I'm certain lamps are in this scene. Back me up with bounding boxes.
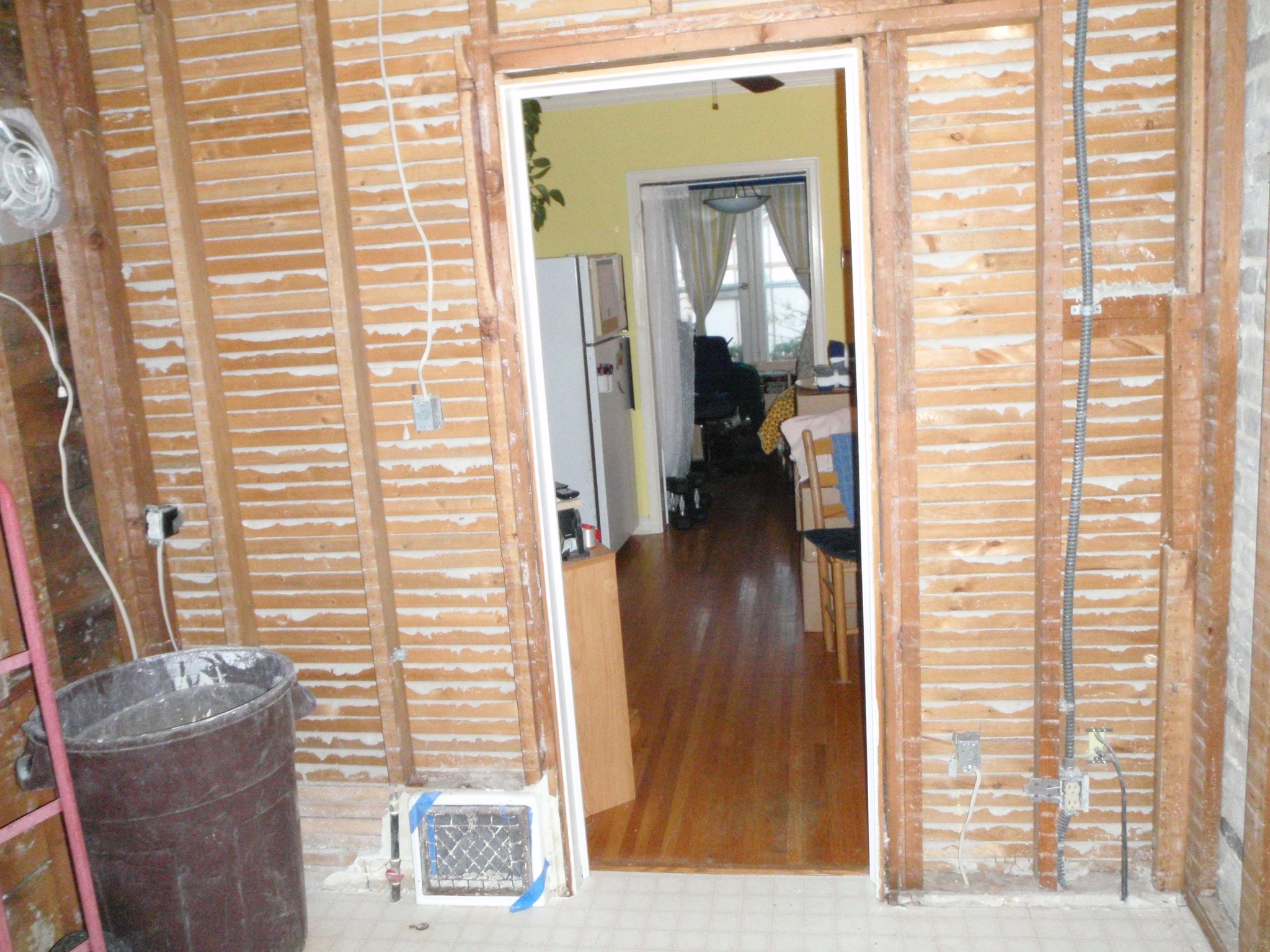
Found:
[703,182,772,214]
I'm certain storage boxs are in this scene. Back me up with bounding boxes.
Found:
[799,483,862,633]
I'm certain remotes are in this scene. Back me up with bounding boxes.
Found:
[556,488,580,501]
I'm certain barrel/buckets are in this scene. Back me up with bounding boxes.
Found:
[16,643,317,950]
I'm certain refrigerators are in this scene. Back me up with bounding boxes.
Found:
[535,251,640,551]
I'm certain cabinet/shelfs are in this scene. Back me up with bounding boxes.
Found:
[562,541,636,819]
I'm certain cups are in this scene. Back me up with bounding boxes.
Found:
[581,524,596,550]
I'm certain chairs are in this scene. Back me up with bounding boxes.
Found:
[802,429,862,680]
[693,335,739,486]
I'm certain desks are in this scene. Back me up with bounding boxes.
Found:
[796,386,857,416]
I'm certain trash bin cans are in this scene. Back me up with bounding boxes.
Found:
[15,645,317,952]
